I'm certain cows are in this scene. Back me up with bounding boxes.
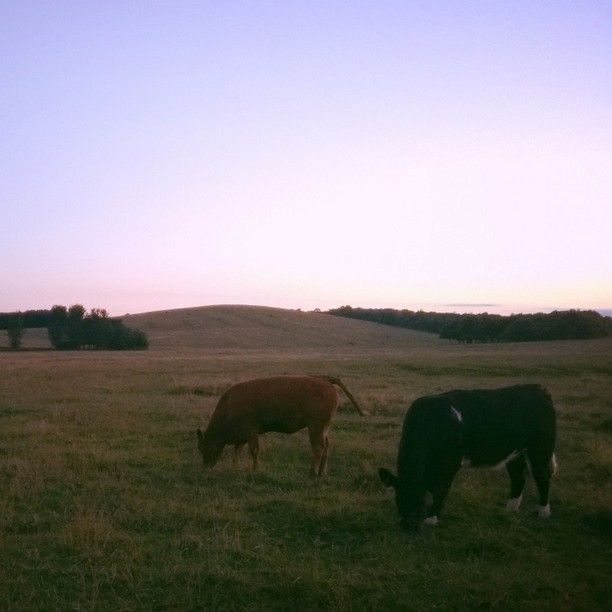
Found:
[381,381,559,527]
[193,371,366,487]
